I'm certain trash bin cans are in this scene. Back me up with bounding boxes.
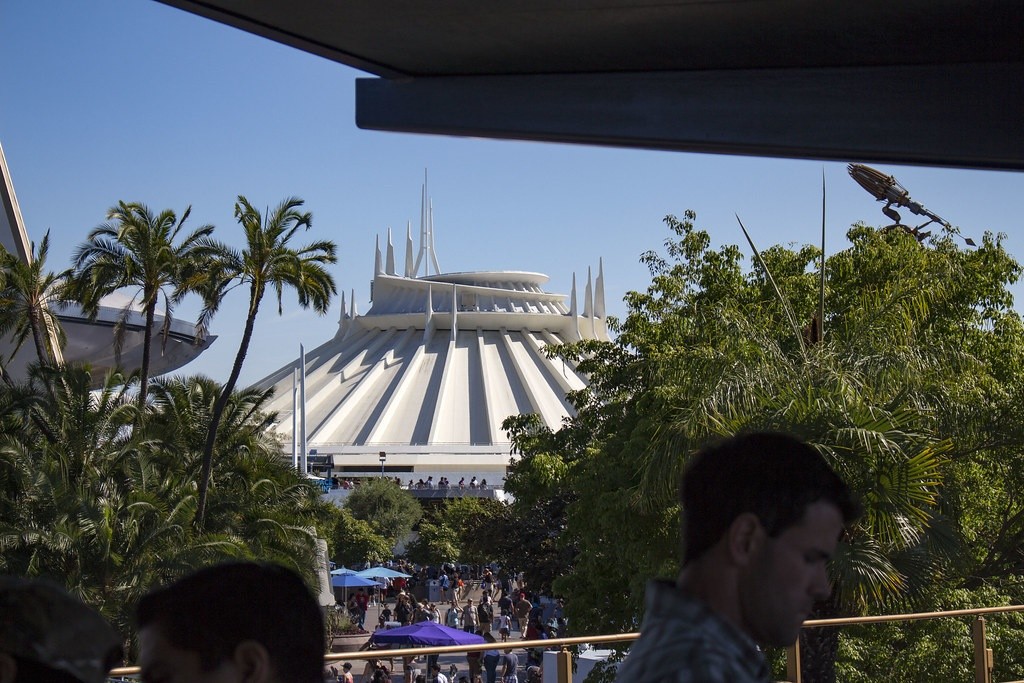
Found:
[428,579,441,602]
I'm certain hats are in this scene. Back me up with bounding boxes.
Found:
[520,593,525,598]
[341,662,352,669]
[0,577,118,683]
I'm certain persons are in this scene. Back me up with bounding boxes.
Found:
[445,599,480,633]
[332,475,488,489]
[375,604,391,631]
[439,571,464,607]
[348,588,368,629]
[133,562,324,682]
[331,663,354,683]
[480,566,510,603]
[616,432,850,683]
[478,589,533,642]
[404,656,417,683]
[393,592,441,627]
[368,577,407,605]
[526,619,550,665]
[432,632,540,683]
[361,660,392,683]
[0,582,120,683]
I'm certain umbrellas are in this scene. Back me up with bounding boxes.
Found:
[332,571,382,601]
[330,565,357,574]
[371,622,482,683]
[359,566,413,577]
